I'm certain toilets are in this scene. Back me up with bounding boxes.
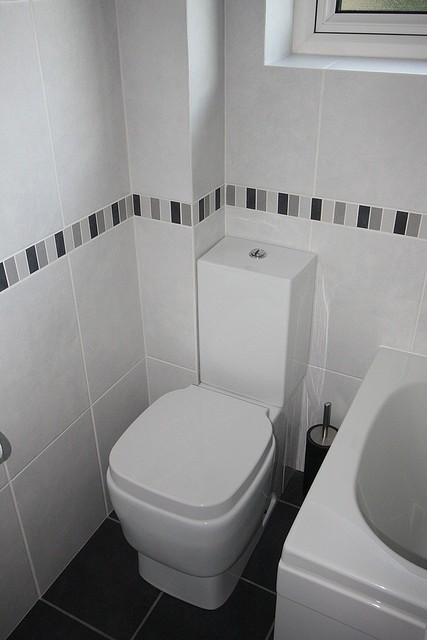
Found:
[106,237,318,610]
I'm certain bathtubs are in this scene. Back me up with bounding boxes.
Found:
[275,348,426,640]
